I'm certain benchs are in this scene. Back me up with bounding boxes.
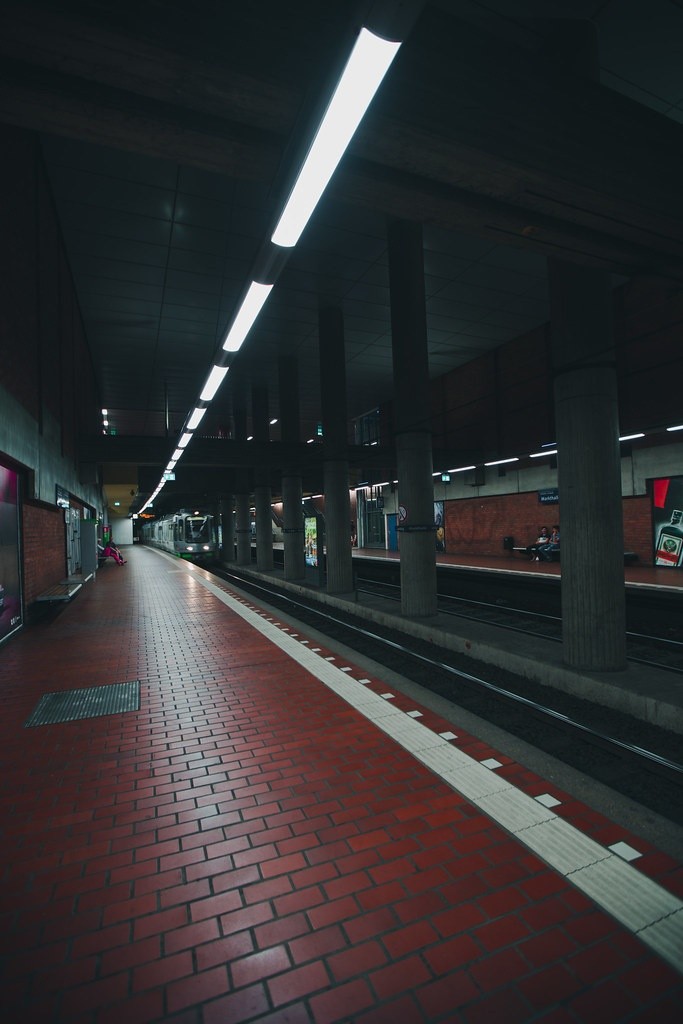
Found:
[59,573,94,584]
[513,547,559,556]
[35,583,83,602]
[623,552,637,562]
[98,555,111,565]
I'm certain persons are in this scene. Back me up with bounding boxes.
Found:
[526,527,550,560]
[98,537,127,565]
[539,525,560,562]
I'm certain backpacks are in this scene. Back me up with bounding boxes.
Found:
[101,546,110,557]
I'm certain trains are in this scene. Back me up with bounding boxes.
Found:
[140,508,212,560]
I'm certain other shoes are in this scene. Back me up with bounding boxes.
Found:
[117,561,124,566]
[353,544,355,547]
[124,560,127,563]
